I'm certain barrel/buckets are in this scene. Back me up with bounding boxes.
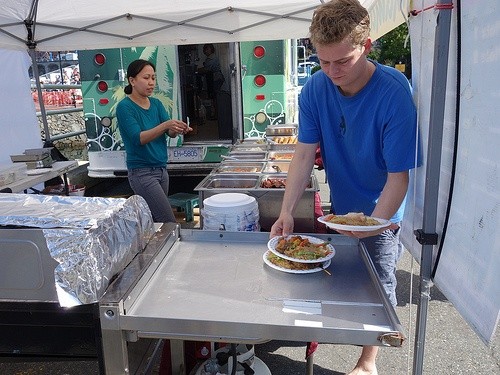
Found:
[201,193,261,232]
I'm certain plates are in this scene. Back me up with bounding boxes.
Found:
[267,234,335,263]
[317,215,391,231]
[264,250,331,273]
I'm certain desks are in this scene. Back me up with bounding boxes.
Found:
[0,161,84,196]
[99,223,407,375]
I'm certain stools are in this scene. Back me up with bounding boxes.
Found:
[167,193,199,222]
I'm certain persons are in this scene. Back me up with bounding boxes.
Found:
[268,0,423,375]
[198,44,226,120]
[115,59,193,222]
